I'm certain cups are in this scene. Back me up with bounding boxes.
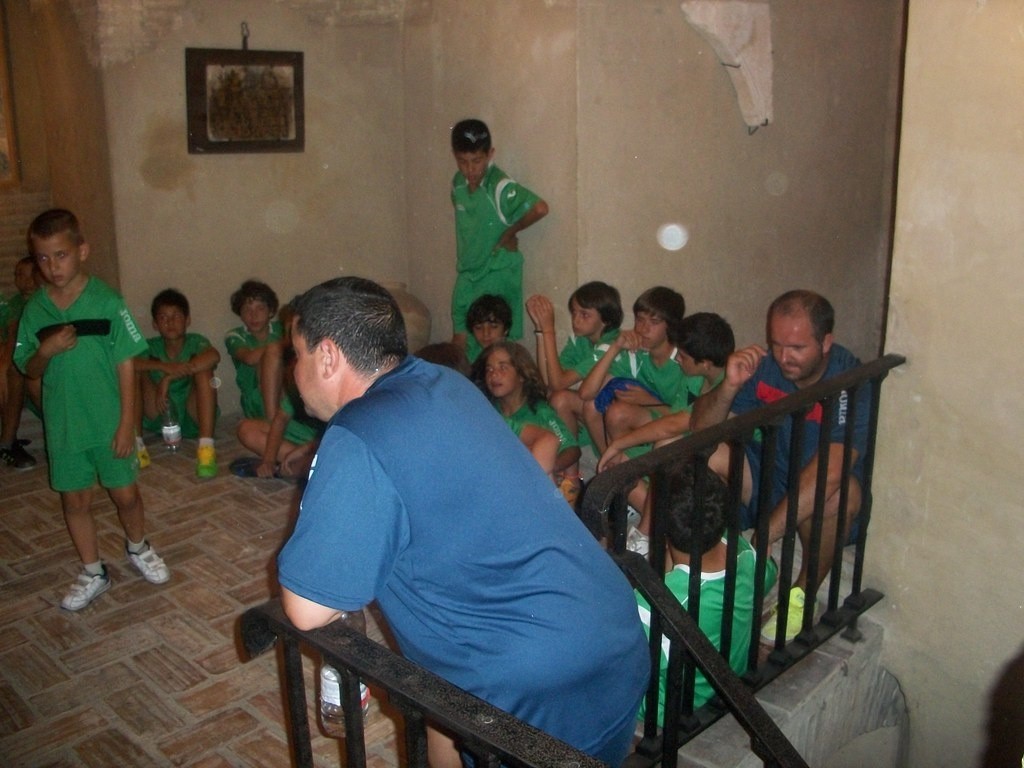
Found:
[196,437,217,479]
[131,430,149,469]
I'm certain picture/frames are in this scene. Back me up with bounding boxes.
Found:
[185,47,306,153]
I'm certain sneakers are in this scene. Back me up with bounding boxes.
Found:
[0,443,38,471]
[556,477,586,504]
[60,565,111,611]
[229,457,286,478]
[132,437,151,467]
[123,539,170,584]
[196,446,219,478]
[625,527,651,558]
[627,504,640,521]
[759,587,819,648]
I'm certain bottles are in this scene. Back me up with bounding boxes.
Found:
[161,402,183,453]
[317,607,369,739]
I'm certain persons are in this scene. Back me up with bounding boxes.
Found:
[625,459,785,730]
[1,256,46,468]
[691,291,873,647]
[468,279,764,564]
[450,118,551,369]
[291,275,654,765]
[128,287,224,480]
[14,207,168,611]
[221,276,328,476]
